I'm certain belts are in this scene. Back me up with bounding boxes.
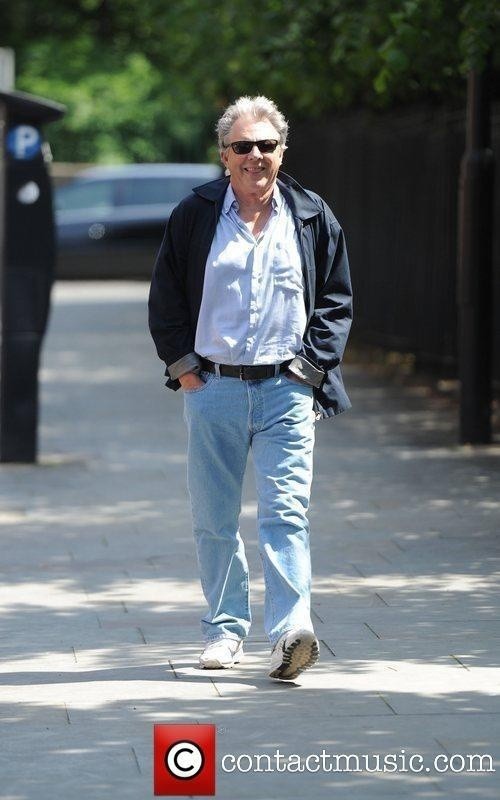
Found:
[198,357,295,381]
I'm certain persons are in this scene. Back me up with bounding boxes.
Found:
[148,96,353,681]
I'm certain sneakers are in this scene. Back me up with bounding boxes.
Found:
[267,628,321,680]
[198,638,245,670]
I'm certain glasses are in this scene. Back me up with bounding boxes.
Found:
[222,138,279,155]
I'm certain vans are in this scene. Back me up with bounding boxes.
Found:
[51,161,224,279]
